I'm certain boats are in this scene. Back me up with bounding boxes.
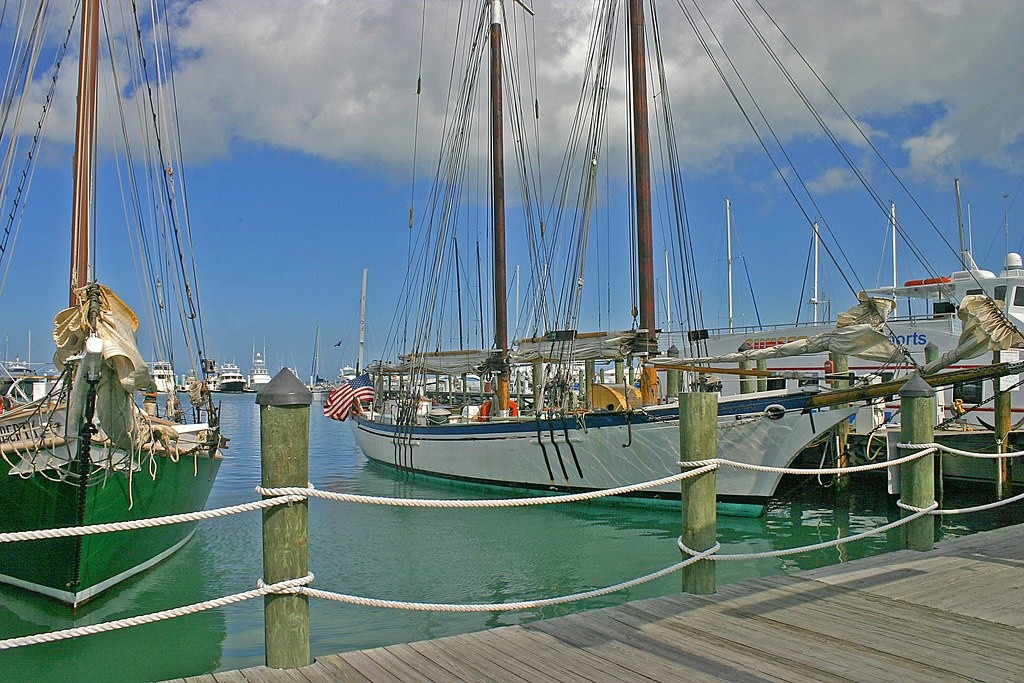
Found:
[242,336,272,393]
[218,355,247,393]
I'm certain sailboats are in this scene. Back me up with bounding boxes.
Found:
[1,1,224,611]
[306,1,1024,519]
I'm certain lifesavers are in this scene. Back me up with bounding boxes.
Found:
[764,403,787,422]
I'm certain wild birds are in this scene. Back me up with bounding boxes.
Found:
[1000,193,1011,199]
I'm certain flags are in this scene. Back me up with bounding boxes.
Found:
[323,373,382,422]
[334,340,342,347]
